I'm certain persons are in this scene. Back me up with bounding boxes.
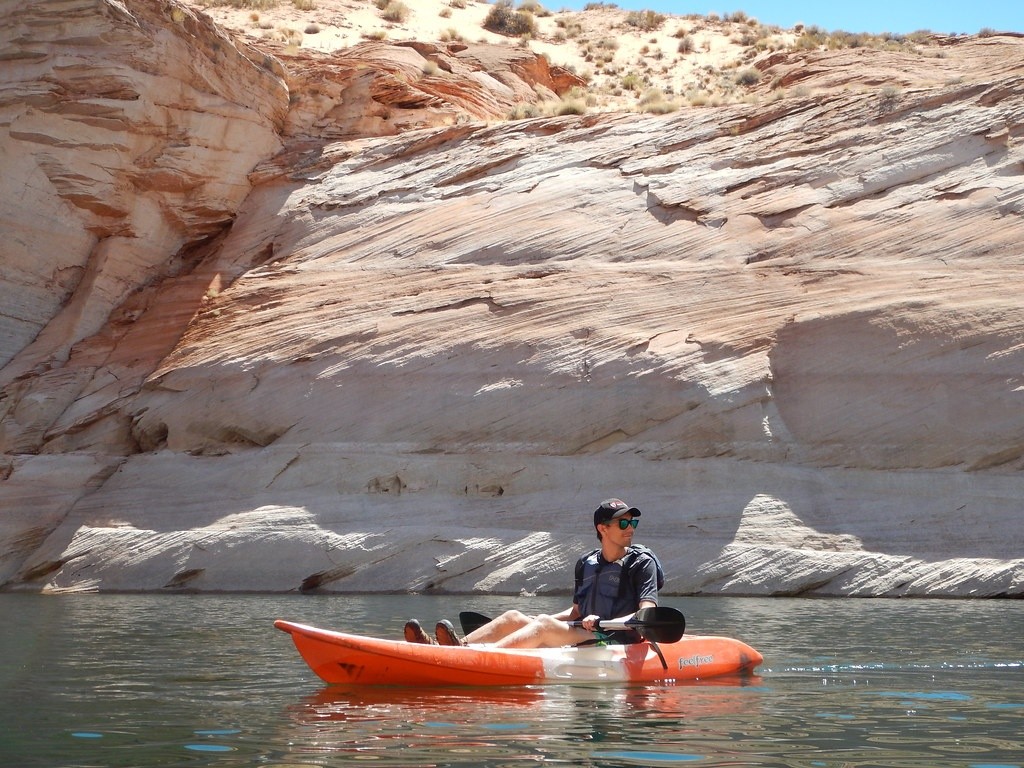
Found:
[403,497,664,648]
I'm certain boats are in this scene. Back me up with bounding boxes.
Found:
[272,619,763,691]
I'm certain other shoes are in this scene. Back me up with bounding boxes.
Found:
[436,618,467,646]
[404,618,436,644]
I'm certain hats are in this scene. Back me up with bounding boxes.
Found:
[594,498,641,526]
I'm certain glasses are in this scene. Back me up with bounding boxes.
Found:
[608,519,639,530]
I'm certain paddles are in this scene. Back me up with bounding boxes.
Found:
[458,606,688,646]
